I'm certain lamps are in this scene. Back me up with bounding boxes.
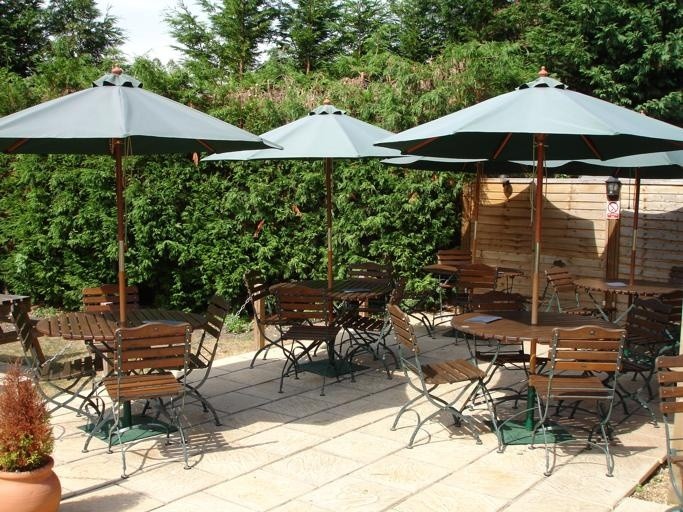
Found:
[604,173,622,201]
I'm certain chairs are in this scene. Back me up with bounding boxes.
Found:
[387,249,682,477]
[243,249,400,396]
[3,281,232,479]
[655,355,683,504]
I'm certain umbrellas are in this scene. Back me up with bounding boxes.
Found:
[372,66,683,432]
[379,151,683,286]
[0,66,283,427]
[201,97,416,365]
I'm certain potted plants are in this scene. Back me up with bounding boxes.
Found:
[0,356,61,512]
